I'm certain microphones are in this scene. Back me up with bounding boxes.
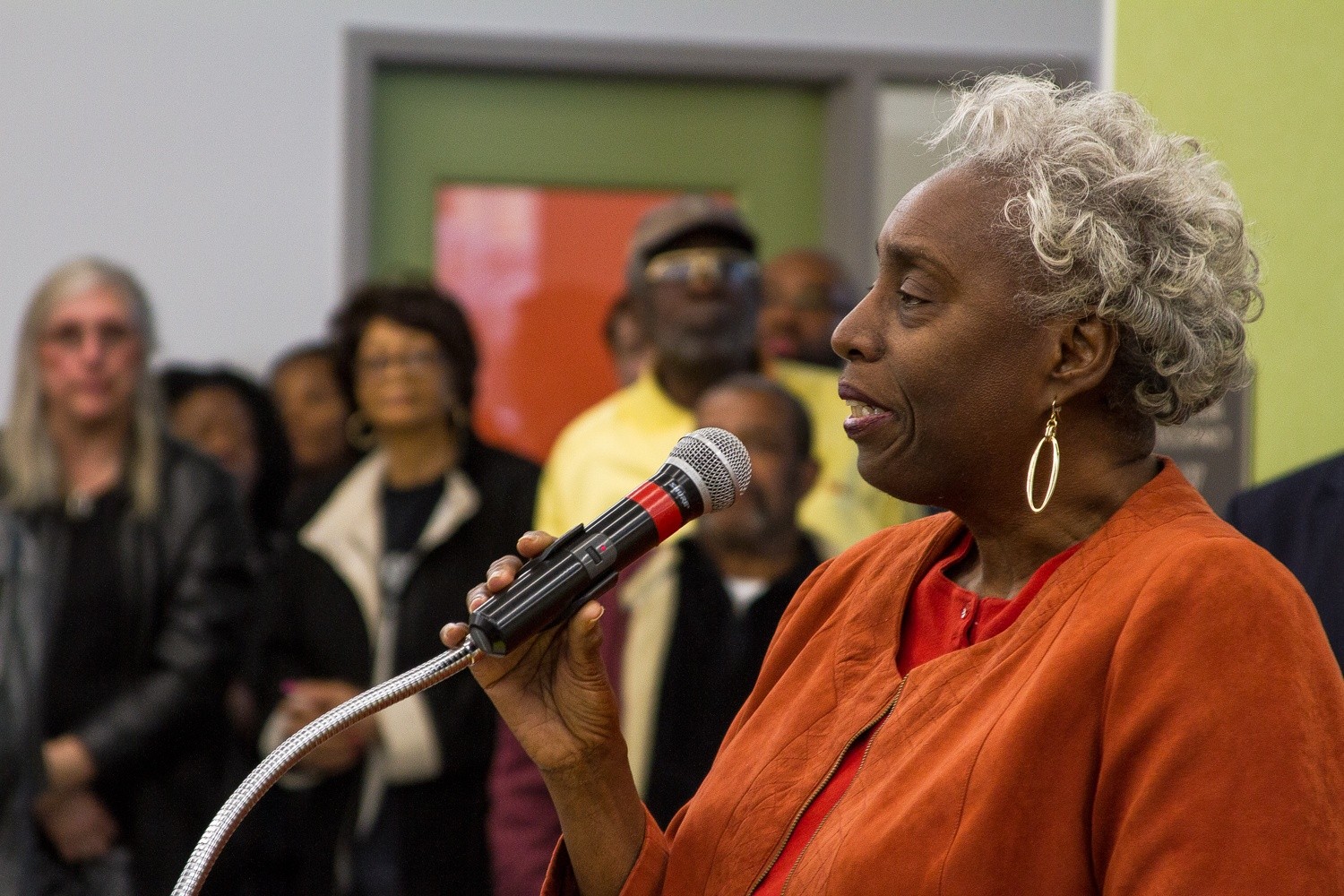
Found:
[468,426,751,656]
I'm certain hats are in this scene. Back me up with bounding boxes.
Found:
[624,203,757,280]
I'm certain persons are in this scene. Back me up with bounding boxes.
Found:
[266,343,366,493]
[0,257,258,896]
[229,268,543,896]
[760,248,857,369]
[533,195,933,565]
[440,64,1344,896]
[488,376,837,896]
[156,362,291,524]
[600,295,645,385]
[1223,451,1344,678]
